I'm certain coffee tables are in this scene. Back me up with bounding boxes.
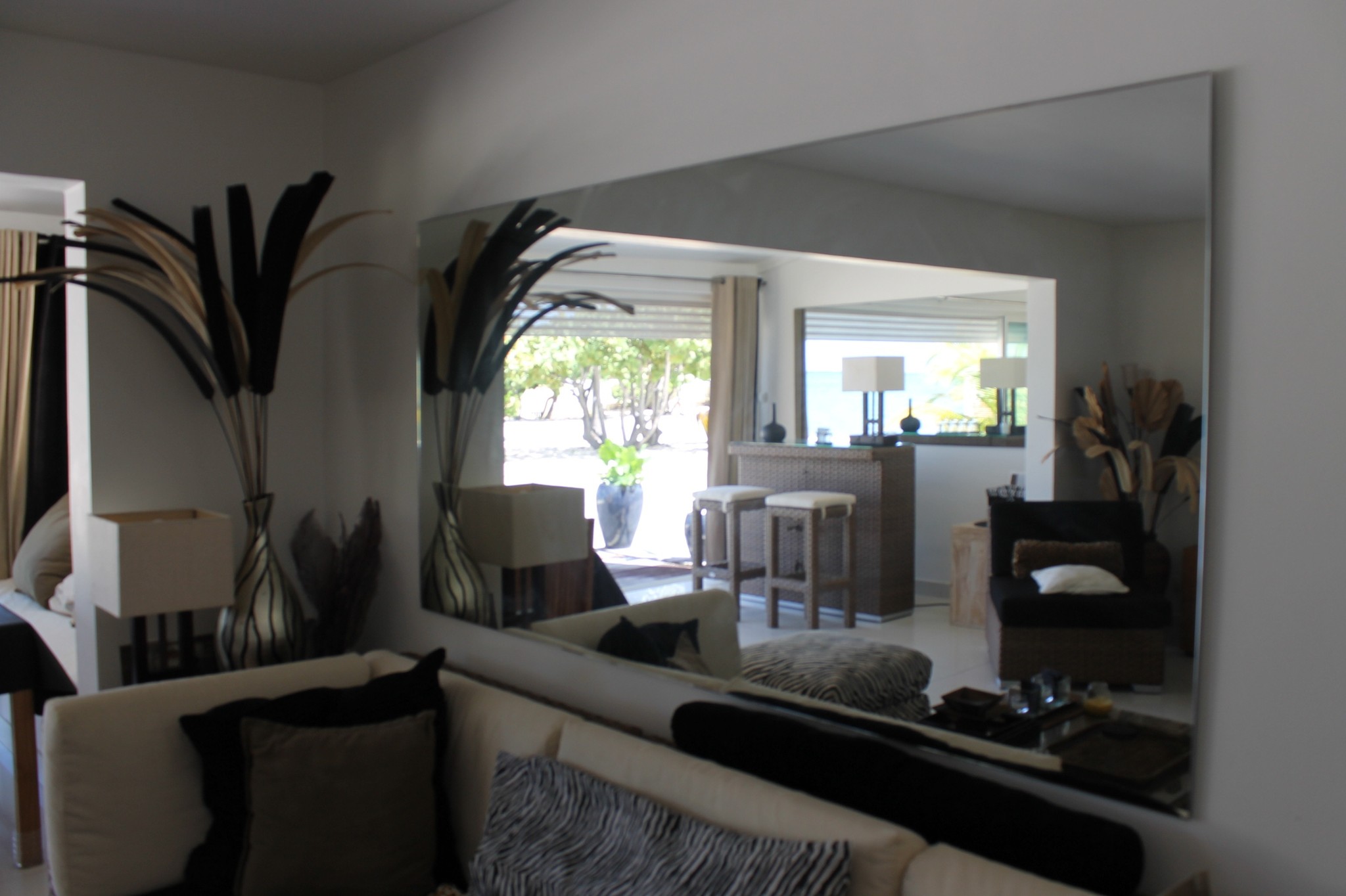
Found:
[934,690,1194,813]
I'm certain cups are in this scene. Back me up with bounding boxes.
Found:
[1006,685,1030,714]
[1053,676,1072,697]
[1029,671,1057,704]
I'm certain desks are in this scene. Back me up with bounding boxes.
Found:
[949,520,993,631]
[724,440,916,623]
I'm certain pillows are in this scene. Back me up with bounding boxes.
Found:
[51,574,75,616]
[601,616,699,665]
[12,494,72,607]
[1029,563,1131,594]
[242,713,438,896]
[868,696,931,722]
[174,647,469,896]
[470,749,855,896]
[742,644,931,707]
[1011,540,1127,580]
[668,631,713,677]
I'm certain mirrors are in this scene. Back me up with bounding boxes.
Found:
[416,74,1216,824]
[794,290,1028,447]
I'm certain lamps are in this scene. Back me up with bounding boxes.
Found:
[841,356,903,444]
[88,508,233,684]
[456,483,586,626]
[980,358,1028,435]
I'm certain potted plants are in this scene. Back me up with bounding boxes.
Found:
[595,443,644,549]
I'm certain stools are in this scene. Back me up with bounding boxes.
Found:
[765,489,857,630]
[691,487,774,623]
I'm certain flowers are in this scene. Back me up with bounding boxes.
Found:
[1061,364,1198,533]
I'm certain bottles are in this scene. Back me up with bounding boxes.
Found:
[900,399,919,432]
[760,402,785,442]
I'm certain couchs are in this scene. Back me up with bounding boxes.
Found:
[506,591,1190,818]
[985,497,1176,686]
[44,648,1145,896]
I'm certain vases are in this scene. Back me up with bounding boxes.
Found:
[760,403,786,443]
[1137,534,1173,628]
[421,483,488,625]
[900,399,920,434]
[220,495,306,668]
